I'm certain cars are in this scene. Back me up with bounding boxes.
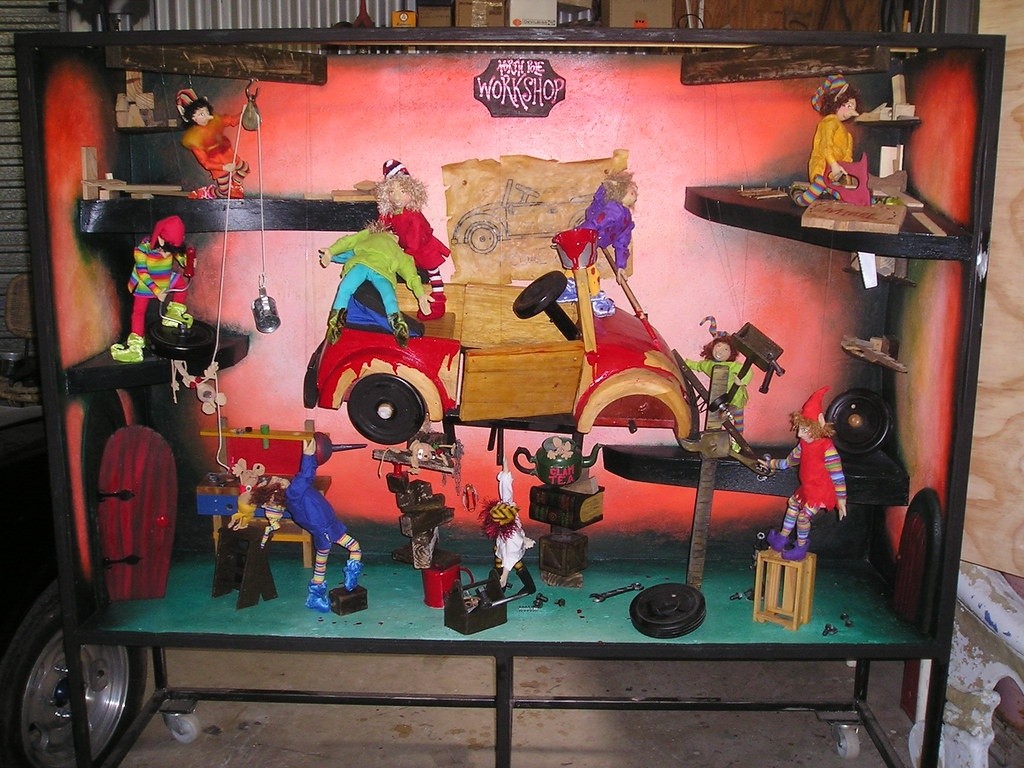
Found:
[299,230,700,453]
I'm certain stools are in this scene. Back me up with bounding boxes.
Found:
[751,548,816,631]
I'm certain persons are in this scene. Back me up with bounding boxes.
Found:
[790,74,860,208]
[109,89,848,614]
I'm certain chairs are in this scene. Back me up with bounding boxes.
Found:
[0,273,40,408]
